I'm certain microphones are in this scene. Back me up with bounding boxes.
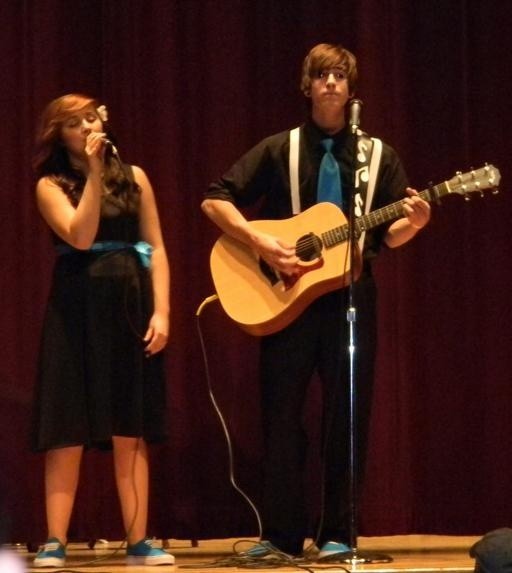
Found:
[347,97,366,136]
[99,135,112,147]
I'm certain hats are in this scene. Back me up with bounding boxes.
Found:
[470,527,512,573]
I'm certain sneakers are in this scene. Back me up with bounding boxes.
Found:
[127,540,175,565]
[33,538,65,567]
[316,541,351,559]
[236,541,281,560]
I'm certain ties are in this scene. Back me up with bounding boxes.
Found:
[317,139,343,209]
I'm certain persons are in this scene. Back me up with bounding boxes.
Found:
[32,94,177,567]
[200,39,431,558]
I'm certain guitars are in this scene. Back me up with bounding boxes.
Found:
[208,161,504,340]
